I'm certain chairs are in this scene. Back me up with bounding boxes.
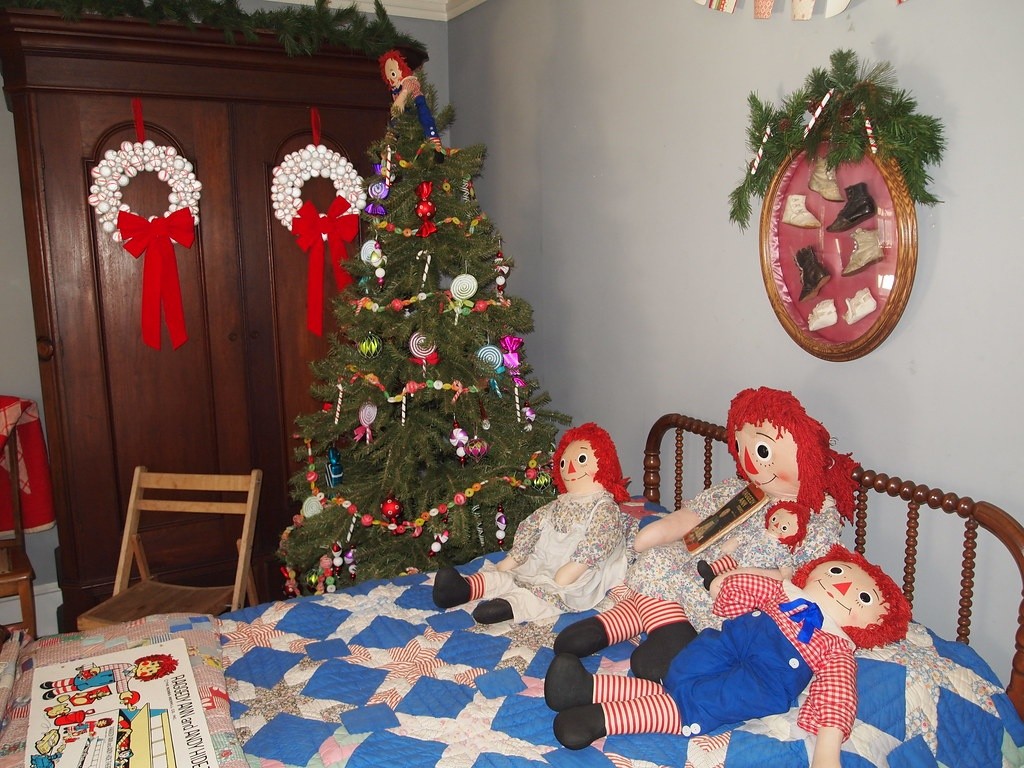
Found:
[0,395,36,642]
[77,466,263,632]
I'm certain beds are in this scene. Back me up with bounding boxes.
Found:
[0,414,1024,768]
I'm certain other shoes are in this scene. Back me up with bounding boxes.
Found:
[808,299,837,331]
[842,288,877,325]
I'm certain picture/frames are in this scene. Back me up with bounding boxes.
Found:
[757,131,920,364]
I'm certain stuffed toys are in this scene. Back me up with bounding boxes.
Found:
[431,384,915,768]
[377,48,447,177]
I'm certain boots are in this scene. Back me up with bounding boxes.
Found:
[793,247,831,303]
[782,195,822,229]
[826,182,877,233]
[808,156,845,201]
[841,228,884,277]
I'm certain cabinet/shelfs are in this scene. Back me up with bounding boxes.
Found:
[0,0,429,635]
